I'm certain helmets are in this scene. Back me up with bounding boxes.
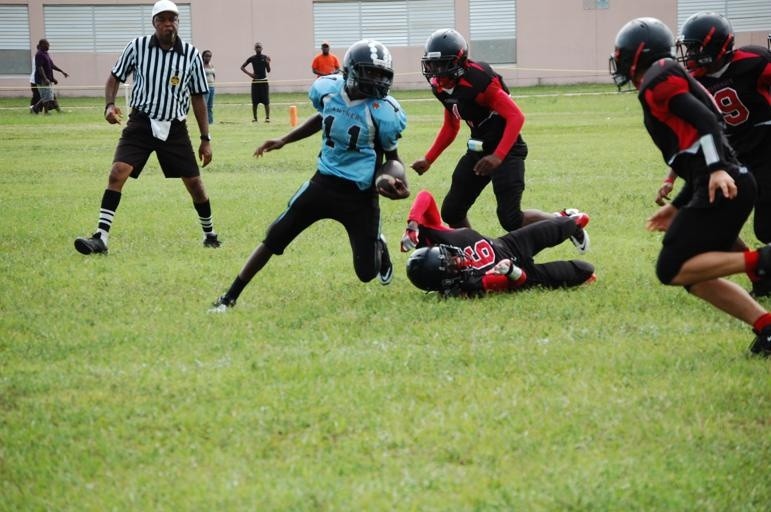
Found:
[343,40,394,99]
[677,12,736,79]
[609,16,677,95]
[406,244,472,295]
[421,28,470,98]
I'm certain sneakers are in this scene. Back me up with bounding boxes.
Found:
[751,246,770,297]
[74,232,108,255]
[378,234,394,286]
[203,235,222,249]
[747,326,770,359]
[561,208,589,254]
[212,294,236,308]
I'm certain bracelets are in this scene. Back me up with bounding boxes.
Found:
[104,103,114,116]
[661,178,674,189]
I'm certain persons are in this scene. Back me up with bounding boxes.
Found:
[312,43,341,78]
[655,12,771,297]
[240,42,271,122]
[208,40,411,312]
[410,28,590,255]
[608,17,771,359]
[401,191,596,299]
[74,0,222,256]
[202,50,221,124]
[29,38,70,114]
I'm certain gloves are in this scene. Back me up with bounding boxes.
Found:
[486,259,513,277]
[399,226,419,251]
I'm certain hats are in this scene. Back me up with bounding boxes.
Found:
[152,0,179,18]
[321,41,330,47]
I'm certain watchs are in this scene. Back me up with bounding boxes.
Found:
[199,132,212,141]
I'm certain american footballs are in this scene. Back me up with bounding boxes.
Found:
[374,159,407,196]
